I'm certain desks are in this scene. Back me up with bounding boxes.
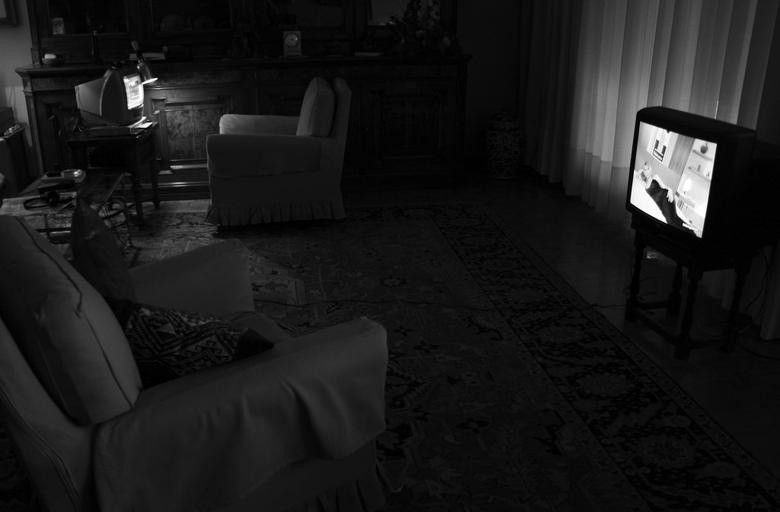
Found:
[623,224,752,362]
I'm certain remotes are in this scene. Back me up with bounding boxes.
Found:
[130,128,144,136]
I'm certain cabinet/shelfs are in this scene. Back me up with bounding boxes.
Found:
[67,129,160,231]
[15,0,472,202]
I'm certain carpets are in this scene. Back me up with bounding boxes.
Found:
[0,203,780,512]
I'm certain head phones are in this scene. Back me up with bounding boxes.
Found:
[23,189,60,211]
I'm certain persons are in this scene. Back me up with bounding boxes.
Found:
[641,161,698,238]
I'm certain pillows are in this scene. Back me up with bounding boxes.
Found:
[0,199,257,422]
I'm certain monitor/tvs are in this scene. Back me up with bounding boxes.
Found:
[622,105,780,267]
[73,63,145,136]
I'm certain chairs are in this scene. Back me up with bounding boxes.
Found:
[0,215,389,512]
[205,76,353,228]
